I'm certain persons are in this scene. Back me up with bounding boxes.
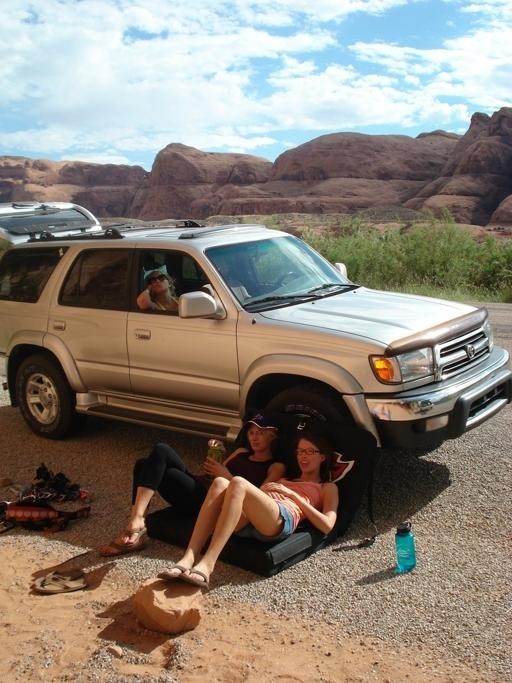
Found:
[135,270,183,313]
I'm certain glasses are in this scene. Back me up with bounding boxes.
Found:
[150,274,166,283]
[295,447,320,456]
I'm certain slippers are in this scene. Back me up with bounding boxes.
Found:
[156,565,210,590]
[33,570,87,593]
[98,526,148,556]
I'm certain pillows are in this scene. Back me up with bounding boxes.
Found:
[143,263,169,284]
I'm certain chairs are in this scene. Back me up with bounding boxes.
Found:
[133,262,179,310]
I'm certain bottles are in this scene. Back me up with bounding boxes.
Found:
[203,444,224,482]
[395,521,416,572]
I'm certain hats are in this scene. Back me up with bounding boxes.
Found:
[143,264,166,280]
[244,411,279,430]
